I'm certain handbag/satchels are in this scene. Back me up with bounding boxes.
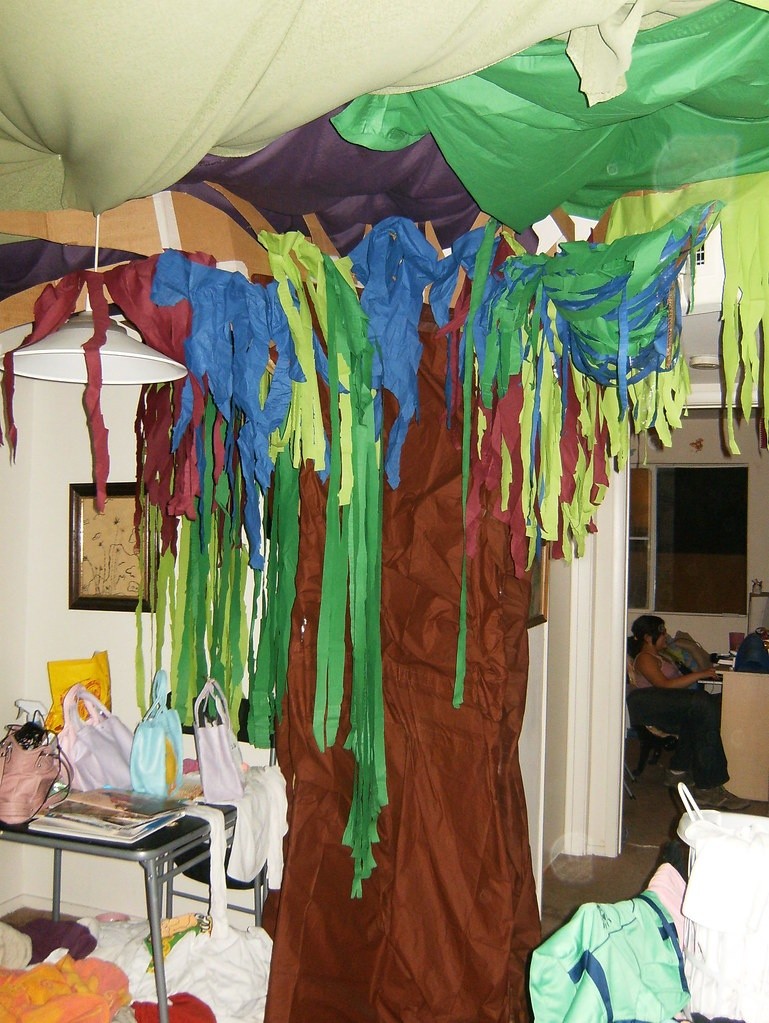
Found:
[129,670,184,798]
[193,678,245,804]
[52,684,134,793]
[0,724,74,824]
[676,782,769,943]
[43,650,113,734]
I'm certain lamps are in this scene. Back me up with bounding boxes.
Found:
[0,214,188,385]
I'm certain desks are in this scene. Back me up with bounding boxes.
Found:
[0,787,269,1023]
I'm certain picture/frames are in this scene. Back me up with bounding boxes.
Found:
[69,482,159,613]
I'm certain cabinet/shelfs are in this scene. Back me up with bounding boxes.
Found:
[714,664,769,803]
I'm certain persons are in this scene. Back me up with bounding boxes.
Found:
[627,615,751,810]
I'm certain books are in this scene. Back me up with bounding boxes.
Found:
[28,788,189,844]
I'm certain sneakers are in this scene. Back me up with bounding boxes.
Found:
[691,785,751,810]
[662,770,696,789]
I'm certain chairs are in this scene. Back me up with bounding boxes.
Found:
[627,653,685,776]
